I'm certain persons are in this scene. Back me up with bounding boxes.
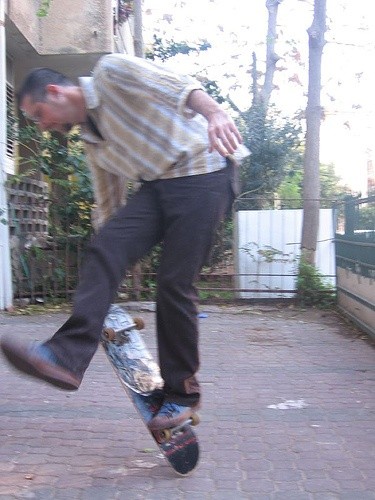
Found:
[0,54,252,430]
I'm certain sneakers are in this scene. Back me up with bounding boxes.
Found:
[1,336,81,390]
[149,387,202,428]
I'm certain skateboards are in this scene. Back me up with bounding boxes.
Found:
[101,304,200,475]
